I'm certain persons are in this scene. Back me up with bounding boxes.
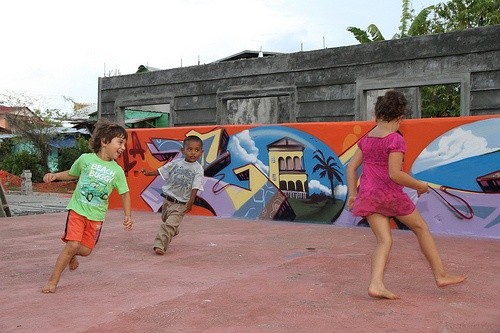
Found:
[42,118,134,294]
[139,135,206,254]
[346,90,469,301]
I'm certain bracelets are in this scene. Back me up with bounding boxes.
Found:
[125,214,131,216]
